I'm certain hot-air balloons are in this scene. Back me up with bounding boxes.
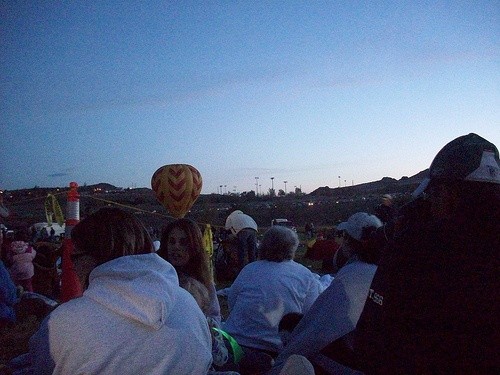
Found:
[151,162,204,219]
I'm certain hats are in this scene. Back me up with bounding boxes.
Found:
[339,212,381,241]
[410,132,500,197]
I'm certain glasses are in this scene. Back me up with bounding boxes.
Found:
[69,252,84,261]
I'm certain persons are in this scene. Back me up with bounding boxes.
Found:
[0,133,500,375]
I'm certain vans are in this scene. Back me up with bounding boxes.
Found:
[28,222,65,241]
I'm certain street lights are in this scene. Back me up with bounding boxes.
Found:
[218,175,347,196]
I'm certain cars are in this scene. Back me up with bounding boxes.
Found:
[278,221,297,232]
